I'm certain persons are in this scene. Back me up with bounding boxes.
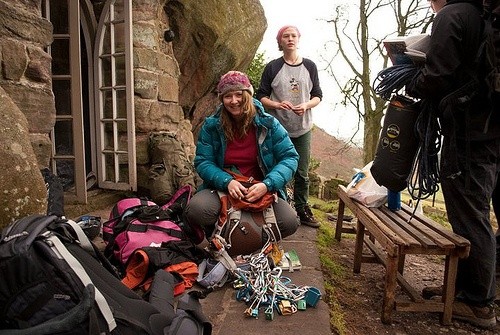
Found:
[256,24,321,228]
[185,70,300,255]
[405,0,500,329]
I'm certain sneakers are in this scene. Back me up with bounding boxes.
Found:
[421,285,496,328]
[295,206,321,227]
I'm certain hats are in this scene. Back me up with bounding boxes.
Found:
[217,70,254,103]
[276,25,301,43]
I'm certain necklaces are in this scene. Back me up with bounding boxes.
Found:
[283,55,298,65]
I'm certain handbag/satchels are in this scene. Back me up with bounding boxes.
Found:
[225,175,275,210]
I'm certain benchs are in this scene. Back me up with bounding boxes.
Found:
[334,184,471,325]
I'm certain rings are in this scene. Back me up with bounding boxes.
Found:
[232,190,236,193]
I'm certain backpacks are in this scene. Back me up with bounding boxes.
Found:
[1,168,213,335]
[370,95,423,193]
[434,0,500,155]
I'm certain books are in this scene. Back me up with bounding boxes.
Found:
[384,33,431,66]
